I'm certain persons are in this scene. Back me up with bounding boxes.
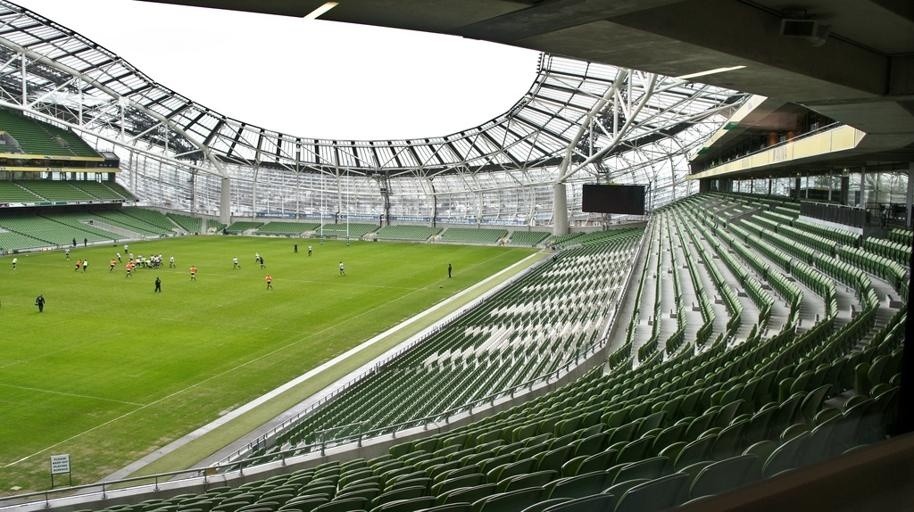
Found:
[232,256,240,271]
[66,237,176,278]
[448,264,452,277]
[154,278,161,293]
[294,243,298,252]
[255,251,266,270]
[190,265,197,280]
[308,245,312,256]
[12,256,18,271]
[265,272,273,289]
[36,294,46,312]
[339,261,344,276]
